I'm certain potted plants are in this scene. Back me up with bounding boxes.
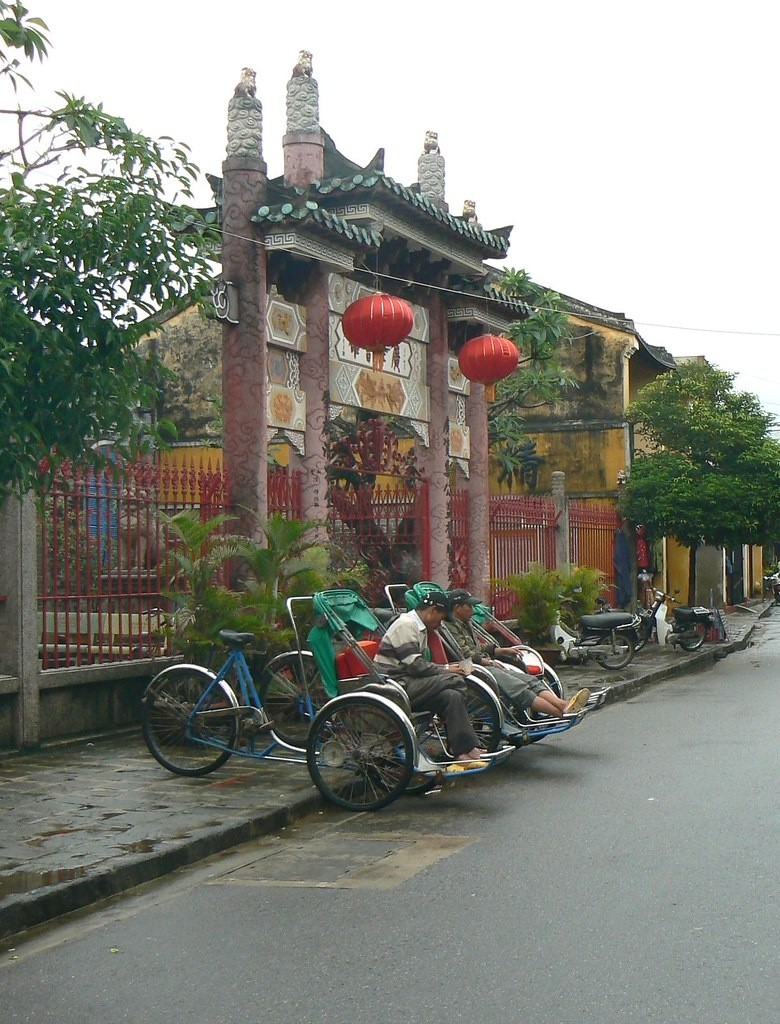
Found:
[227,502,334,735]
[140,509,288,747]
[488,561,571,668]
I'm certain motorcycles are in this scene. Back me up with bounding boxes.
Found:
[623,567,712,656]
[520,586,642,673]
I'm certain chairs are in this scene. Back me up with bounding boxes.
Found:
[405,581,528,701]
[306,589,434,725]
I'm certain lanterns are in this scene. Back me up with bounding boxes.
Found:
[343,292,415,373]
[457,333,519,402]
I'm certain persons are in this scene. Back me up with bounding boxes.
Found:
[442,590,589,719]
[371,592,493,773]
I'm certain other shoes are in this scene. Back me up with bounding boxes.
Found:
[563,688,590,713]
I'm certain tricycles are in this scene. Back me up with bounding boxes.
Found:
[138,582,613,810]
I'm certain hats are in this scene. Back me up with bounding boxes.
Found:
[448,589,482,603]
[421,592,454,623]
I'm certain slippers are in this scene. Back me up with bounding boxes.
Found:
[461,761,489,769]
[433,764,467,774]
